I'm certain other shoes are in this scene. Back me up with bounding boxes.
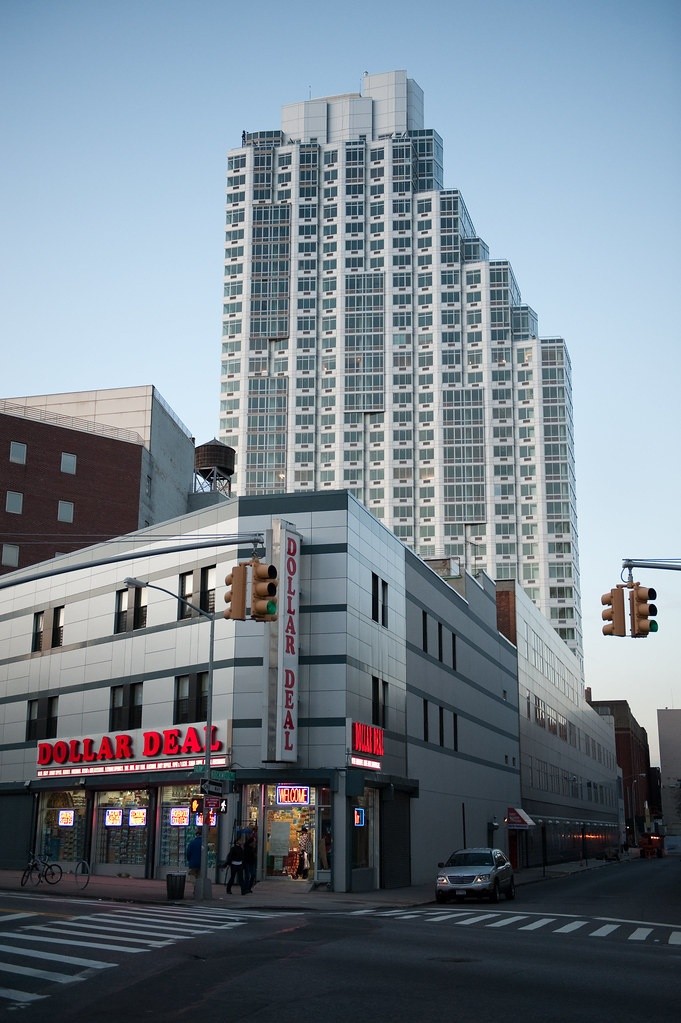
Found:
[241,887,245,895]
[226,886,232,894]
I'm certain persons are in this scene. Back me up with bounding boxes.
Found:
[186,832,202,899]
[242,823,254,833]
[227,837,257,895]
[623,841,629,856]
[291,827,312,880]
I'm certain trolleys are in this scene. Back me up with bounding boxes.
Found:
[283,851,301,880]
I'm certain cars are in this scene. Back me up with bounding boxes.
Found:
[436,847,515,905]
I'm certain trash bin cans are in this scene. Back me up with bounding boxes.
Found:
[166,873,186,900]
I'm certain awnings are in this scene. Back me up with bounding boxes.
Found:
[507,807,536,830]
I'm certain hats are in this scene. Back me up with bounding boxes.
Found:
[195,830,201,835]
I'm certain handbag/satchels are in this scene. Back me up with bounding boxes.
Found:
[304,851,310,870]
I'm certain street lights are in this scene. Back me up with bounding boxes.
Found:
[123,575,215,902]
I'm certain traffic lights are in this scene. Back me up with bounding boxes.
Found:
[219,799,227,813]
[224,566,247,621]
[601,589,626,637]
[251,562,278,623]
[190,798,204,813]
[629,584,658,638]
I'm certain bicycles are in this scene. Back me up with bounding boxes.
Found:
[21,849,64,886]
